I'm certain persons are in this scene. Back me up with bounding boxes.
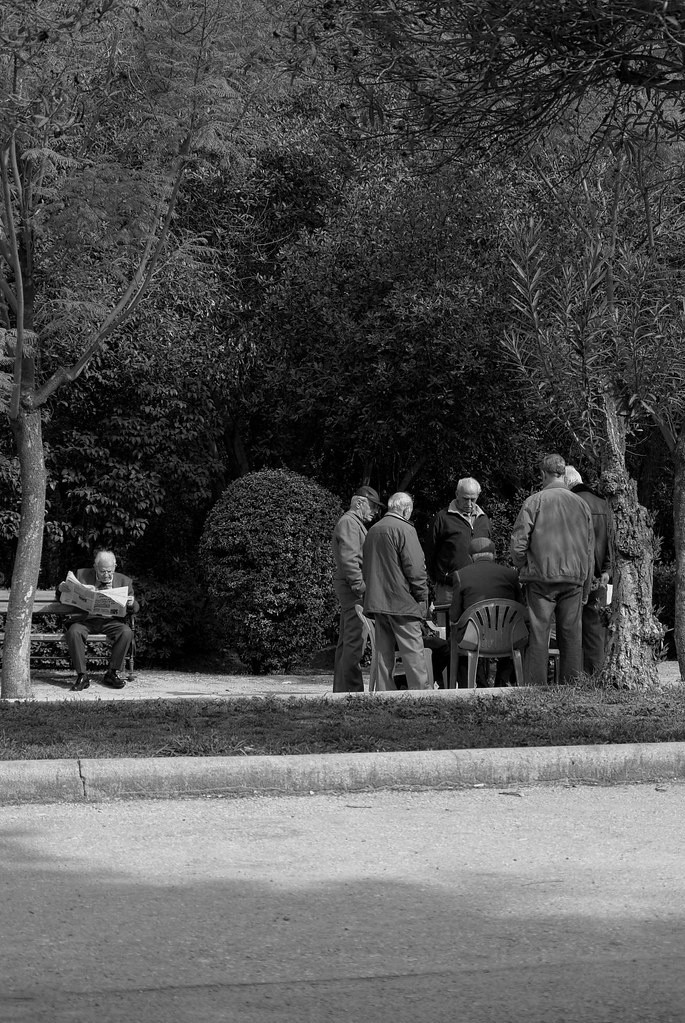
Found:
[334,485,450,694]
[511,453,614,686]
[55,549,140,691]
[427,477,523,687]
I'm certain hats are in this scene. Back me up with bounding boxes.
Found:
[356,486,387,509]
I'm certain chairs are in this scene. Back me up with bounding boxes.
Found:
[355,604,434,691]
[448,598,531,688]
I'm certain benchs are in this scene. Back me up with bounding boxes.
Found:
[0,590,136,681]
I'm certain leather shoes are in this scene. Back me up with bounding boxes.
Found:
[72,675,90,691]
[104,673,126,687]
